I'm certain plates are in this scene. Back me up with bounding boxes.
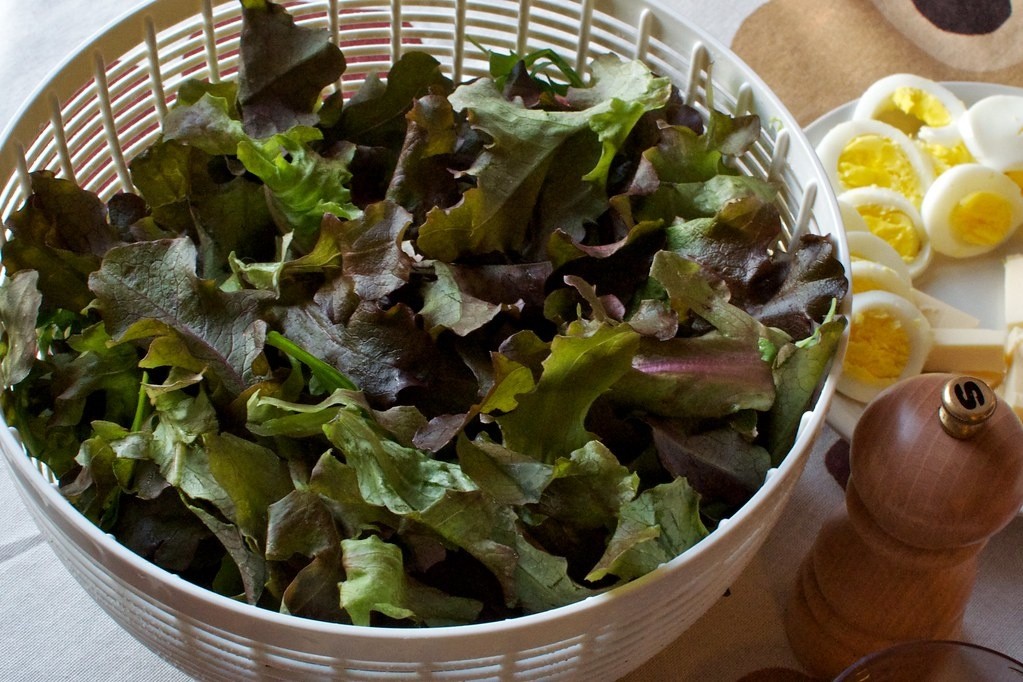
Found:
[803,82,1023,515]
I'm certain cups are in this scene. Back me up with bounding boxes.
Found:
[833,640,1023,682]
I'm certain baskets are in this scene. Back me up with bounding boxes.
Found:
[0,0,852,682]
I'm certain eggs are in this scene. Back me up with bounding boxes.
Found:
[816,73,1023,403]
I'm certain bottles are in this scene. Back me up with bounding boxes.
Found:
[782,372,1023,682]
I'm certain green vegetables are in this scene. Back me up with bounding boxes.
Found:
[0,0,851,630]
